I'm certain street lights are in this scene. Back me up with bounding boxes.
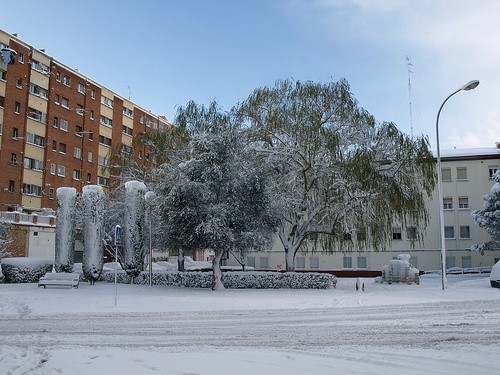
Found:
[143,191,156,286]
[435,80,479,290]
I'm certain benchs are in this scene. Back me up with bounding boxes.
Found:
[38,273,80,290]
